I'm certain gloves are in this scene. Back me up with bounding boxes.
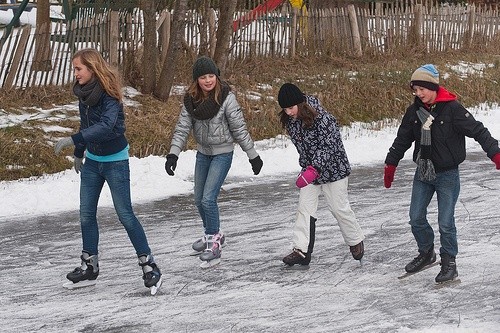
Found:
[54,136,74,158]
[296,166,319,189]
[491,151,500,170]
[73,156,84,173]
[384,164,395,188]
[249,154,263,176]
[164,154,178,176]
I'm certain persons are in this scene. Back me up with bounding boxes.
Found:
[165,56,263,269]
[275,83,365,270]
[382,64,500,285]
[55,47,163,295]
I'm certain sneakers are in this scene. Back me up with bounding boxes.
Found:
[405,243,436,273]
[137,254,162,295]
[191,230,227,256]
[199,235,221,268]
[280,249,311,272]
[350,241,364,265]
[62,251,100,289]
[435,252,456,282]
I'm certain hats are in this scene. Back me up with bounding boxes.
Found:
[278,82,305,107]
[410,65,440,91]
[192,57,218,81]
